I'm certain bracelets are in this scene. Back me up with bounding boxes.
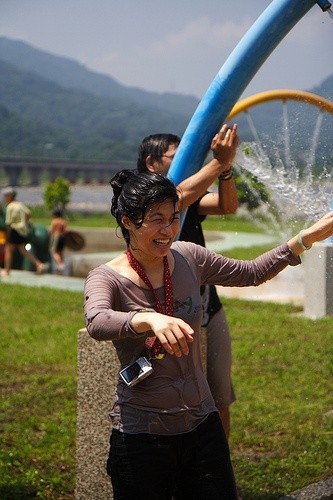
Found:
[297,230,312,251]
[218,167,234,180]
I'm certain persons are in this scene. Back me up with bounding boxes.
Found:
[81,167,333,499]
[44,208,67,275]
[136,122,240,441]
[0,188,47,277]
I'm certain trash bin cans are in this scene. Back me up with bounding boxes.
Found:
[22,225,51,273]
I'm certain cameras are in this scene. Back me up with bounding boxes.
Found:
[119,356,154,387]
